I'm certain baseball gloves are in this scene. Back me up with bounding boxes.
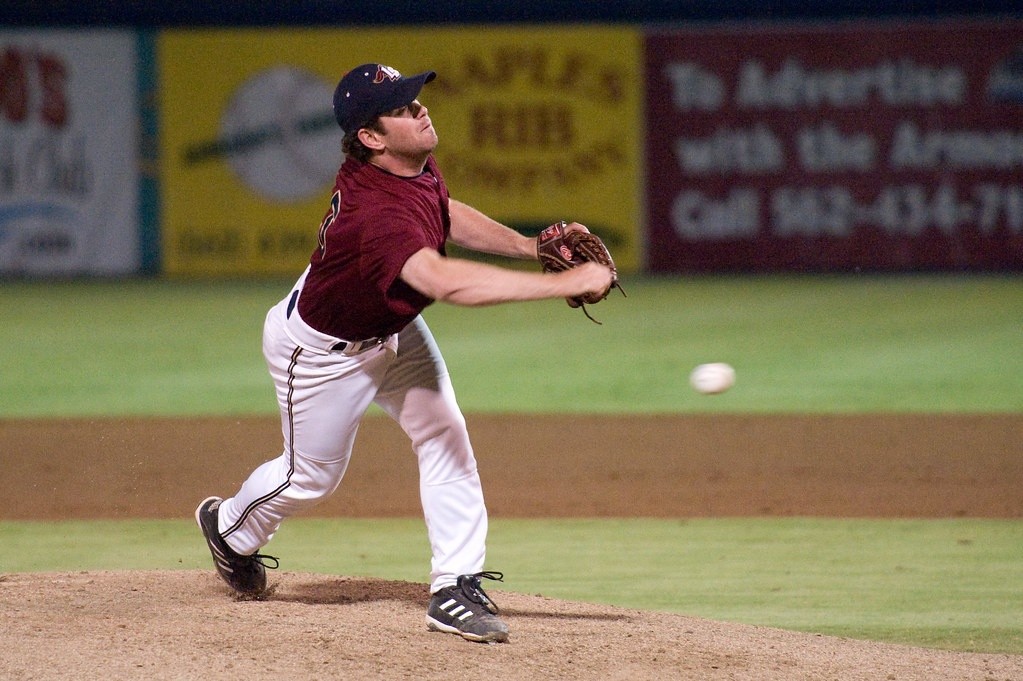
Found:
[535,219,617,306]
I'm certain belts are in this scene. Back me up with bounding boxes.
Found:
[286,290,392,352]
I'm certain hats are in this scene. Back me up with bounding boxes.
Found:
[334,63,436,135]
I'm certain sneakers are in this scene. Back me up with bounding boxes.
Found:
[424,571,509,642]
[195,496,279,594]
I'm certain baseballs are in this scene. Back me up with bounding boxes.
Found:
[689,362,736,395]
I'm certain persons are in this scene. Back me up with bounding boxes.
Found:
[195,62,618,642]
